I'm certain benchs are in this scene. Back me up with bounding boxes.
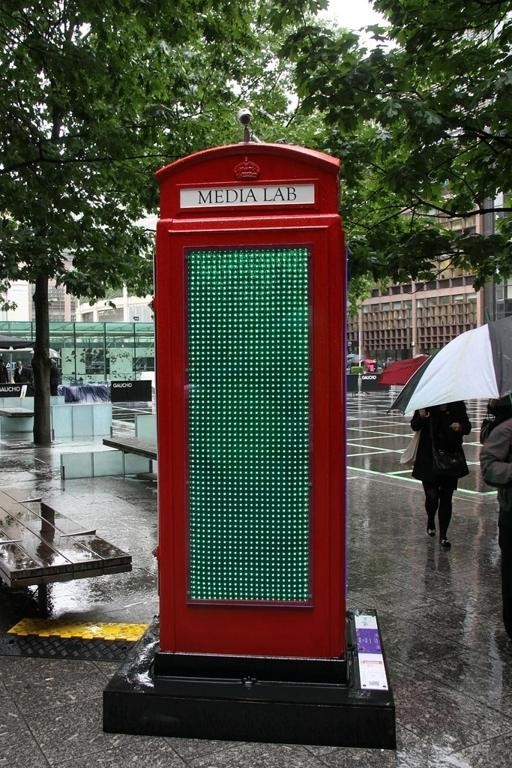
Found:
[0,487,132,617]
[104,438,157,482]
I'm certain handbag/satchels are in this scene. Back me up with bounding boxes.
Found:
[429,443,471,487]
[478,413,495,445]
[399,428,422,466]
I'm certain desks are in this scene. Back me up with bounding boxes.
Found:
[0,407,34,418]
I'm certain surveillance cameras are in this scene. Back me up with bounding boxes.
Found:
[238,107,253,141]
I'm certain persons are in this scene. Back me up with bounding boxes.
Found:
[14,359,34,383]
[0,359,12,384]
[479,395,511,443]
[399,399,473,545]
[363,356,392,373]
[478,418,512,637]
[50,358,62,396]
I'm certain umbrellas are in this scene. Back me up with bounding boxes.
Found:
[387,314,511,417]
[346,352,357,358]
[376,352,429,418]
[30,347,62,359]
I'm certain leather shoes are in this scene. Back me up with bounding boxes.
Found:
[438,535,451,549]
[426,518,437,537]
